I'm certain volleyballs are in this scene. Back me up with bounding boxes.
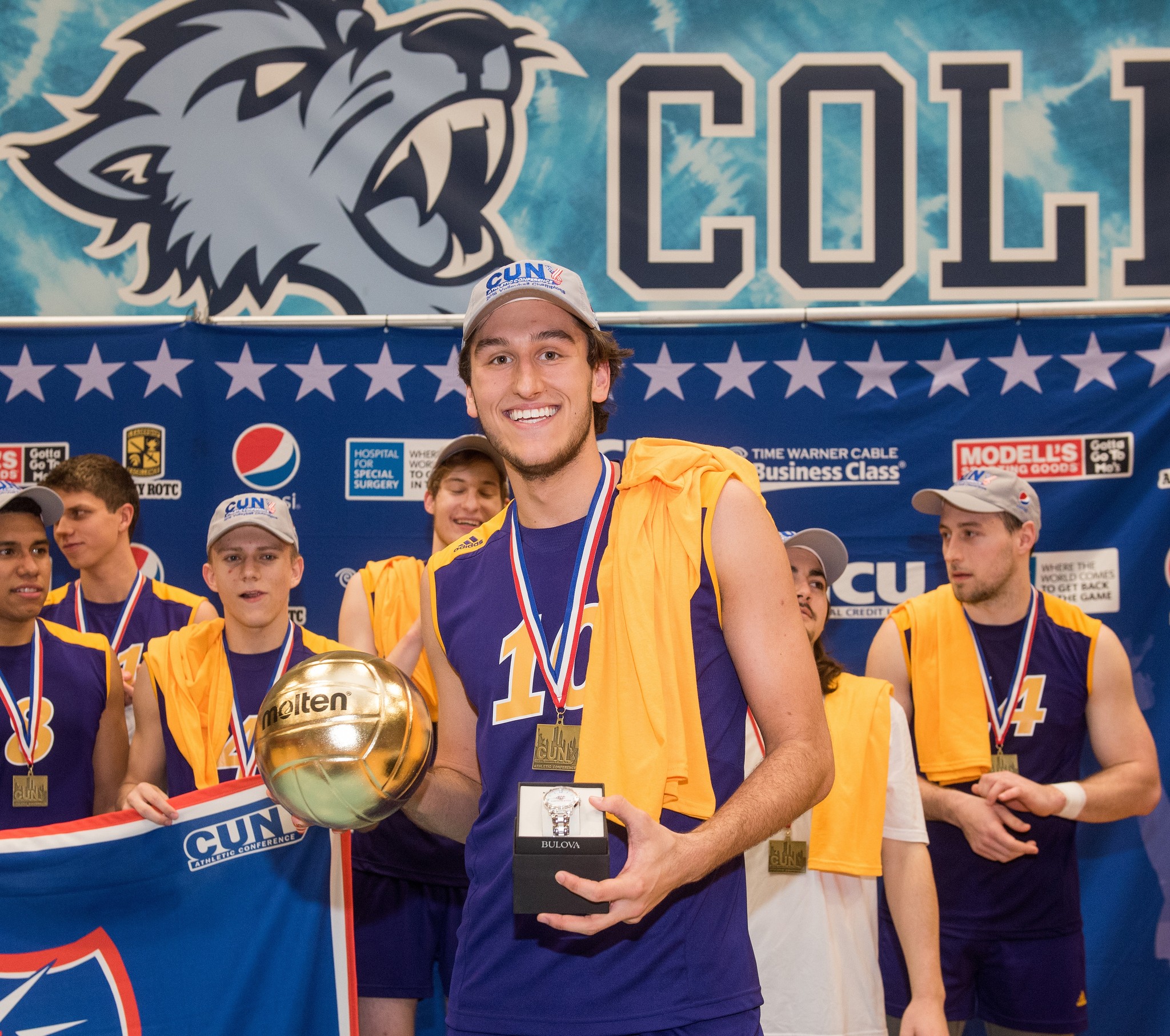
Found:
[255,649,437,830]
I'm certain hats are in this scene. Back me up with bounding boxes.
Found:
[460,260,601,347]
[206,492,299,559]
[910,468,1042,540]
[779,528,848,590]
[0,480,64,528]
[429,433,510,491]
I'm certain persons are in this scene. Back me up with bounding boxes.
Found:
[0,433,1161,1036]
[401,261,837,1036]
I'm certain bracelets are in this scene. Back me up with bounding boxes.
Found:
[1051,780,1088,820]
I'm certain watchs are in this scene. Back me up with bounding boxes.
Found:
[543,787,581,837]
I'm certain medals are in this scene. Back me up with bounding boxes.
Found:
[532,714,581,771]
[769,840,805,874]
[991,754,1018,774]
[12,775,49,808]
